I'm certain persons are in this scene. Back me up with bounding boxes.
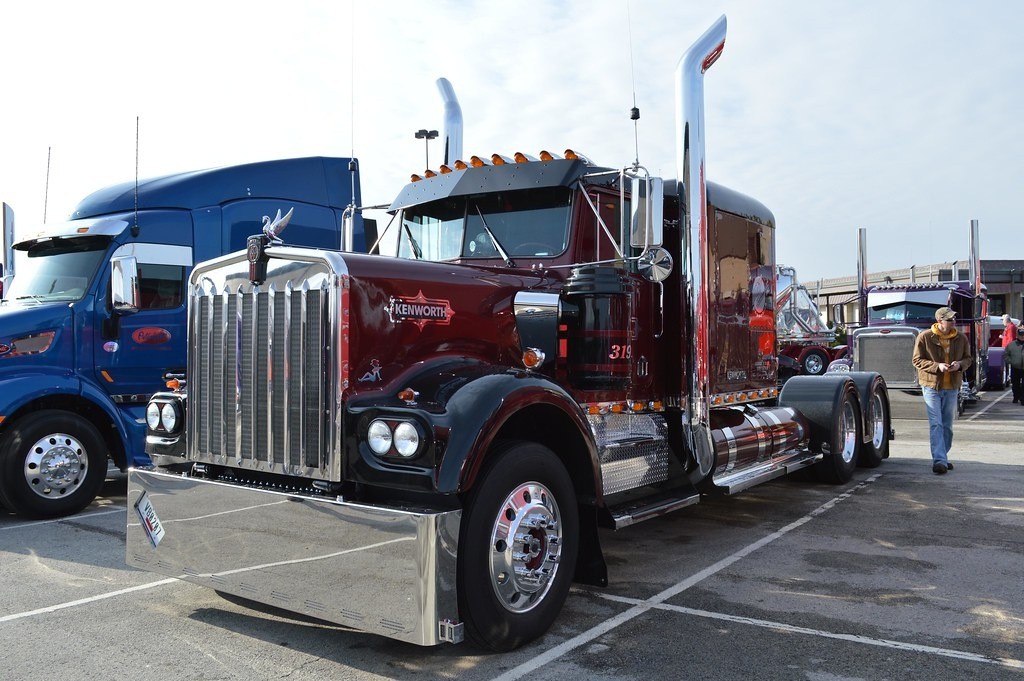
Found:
[999,314,1024,406]
[912,307,973,474]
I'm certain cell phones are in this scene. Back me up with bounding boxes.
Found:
[948,365,954,368]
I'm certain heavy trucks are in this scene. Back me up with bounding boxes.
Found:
[850,220,1019,422]
[127,12,897,652]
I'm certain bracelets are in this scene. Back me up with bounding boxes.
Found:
[957,365,962,373]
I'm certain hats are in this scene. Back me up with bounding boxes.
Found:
[999,314,1010,320]
[1018,329,1024,334]
[935,307,958,320]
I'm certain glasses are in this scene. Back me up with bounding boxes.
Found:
[947,316,956,321]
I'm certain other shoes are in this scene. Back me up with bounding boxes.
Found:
[947,463,953,470]
[1013,398,1018,403]
[1022,401,1024,405]
[933,463,948,474]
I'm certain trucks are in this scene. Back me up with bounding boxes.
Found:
[0,156,380,522]
